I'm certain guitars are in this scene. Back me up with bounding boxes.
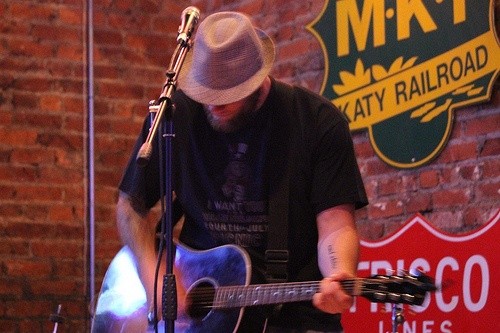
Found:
[90,231,438,333]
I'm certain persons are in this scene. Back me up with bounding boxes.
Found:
[113,10,369,333]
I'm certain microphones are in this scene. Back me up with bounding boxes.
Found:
[176,6,201,45]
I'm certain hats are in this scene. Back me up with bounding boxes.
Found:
[178,12,275,106]
